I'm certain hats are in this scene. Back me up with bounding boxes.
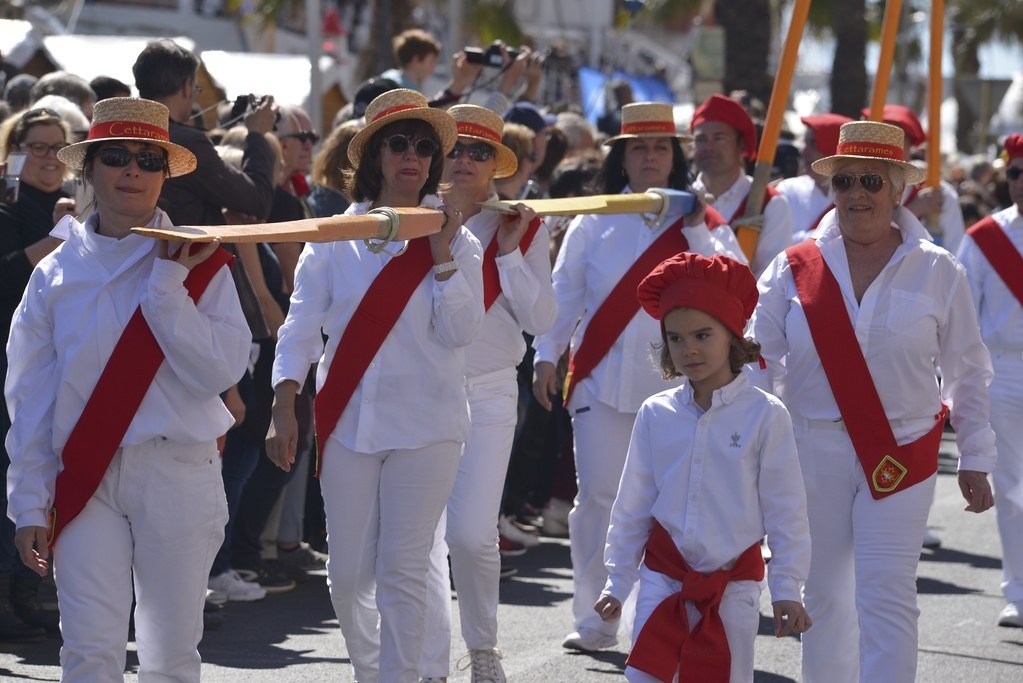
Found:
[604,102,695,144]
[445,105,518,178]
[56,96,197,179]
[504,103,557,130]
[802,103,922,152]
[637,252,760,339]
[810,121,925,185]
[689,95,756,161]
[347,88,458,168]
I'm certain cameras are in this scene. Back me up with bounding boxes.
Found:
[232,95,282,132]
[465,40,520,68]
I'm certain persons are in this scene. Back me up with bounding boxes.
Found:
[942,160,1013,228]
[265,88,486,683]
[595,250,815,682]
[3,98,253,683]
[529,104,754,653]
[777,112,854,236]
[303,33,804,597]
[742,121,996,683]
[0,36,325,614]
[955,133,1023,626]
[689,92,791,398]
[861,103,968,254]
[419,104,563,683]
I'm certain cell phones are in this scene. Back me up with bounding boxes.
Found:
[74,177,96,223]
[4,152,28,204]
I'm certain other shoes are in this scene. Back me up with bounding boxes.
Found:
[497,502,569,575]
[999,603,1023,627]
[923,532,941,548]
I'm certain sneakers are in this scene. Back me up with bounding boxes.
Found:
[563,627,618,651]
[202,541,328,622]
[456,648,507,683]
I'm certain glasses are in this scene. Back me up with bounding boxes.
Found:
[308,132,318,144]
[193,85,201,95]
[1005,167,1023,180]
[447,141,494,162]
[287,131,307,142]
[20,142,70,158]
[831,172,889,193]
[527,152,538,160]
[74,130,87,141]
[381,134,439,157]
[90,148,166,172]
[22,107,61,121]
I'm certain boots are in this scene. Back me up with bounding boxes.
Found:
[12,571,59,637]
[1,570,47,644]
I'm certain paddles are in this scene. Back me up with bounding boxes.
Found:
[867,0,903,123]
[737,0,812,269]
[130,187,715,243]
[927,0,943,225]
